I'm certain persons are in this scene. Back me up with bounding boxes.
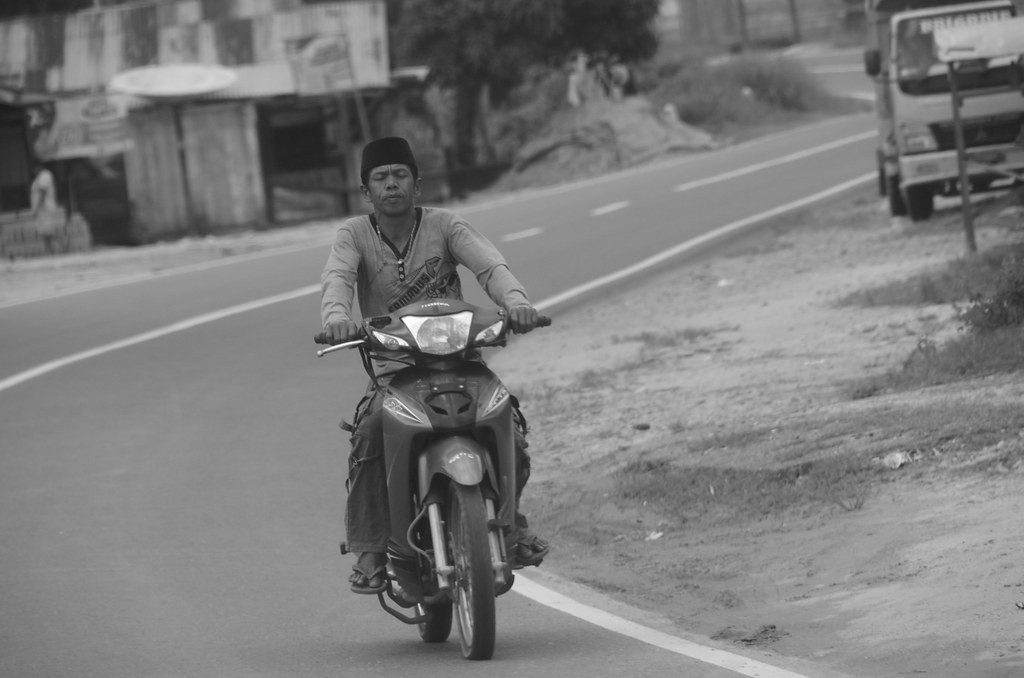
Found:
[29,155,66,254]
[320,137,550,594]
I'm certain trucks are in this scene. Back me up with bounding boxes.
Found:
[864,2,1024,219]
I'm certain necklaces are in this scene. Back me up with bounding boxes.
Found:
[377,221,416,265]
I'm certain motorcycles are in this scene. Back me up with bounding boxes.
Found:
[315,316,553,659]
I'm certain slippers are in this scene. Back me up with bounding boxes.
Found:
[348,565,387,594]
[517,535,550,565]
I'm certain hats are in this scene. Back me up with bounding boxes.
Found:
[360,137,418,177]
